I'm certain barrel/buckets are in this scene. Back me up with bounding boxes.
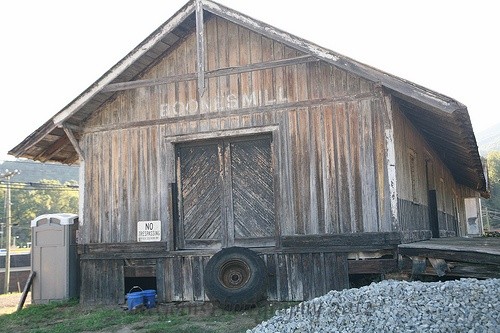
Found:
[125,286,157,311]
